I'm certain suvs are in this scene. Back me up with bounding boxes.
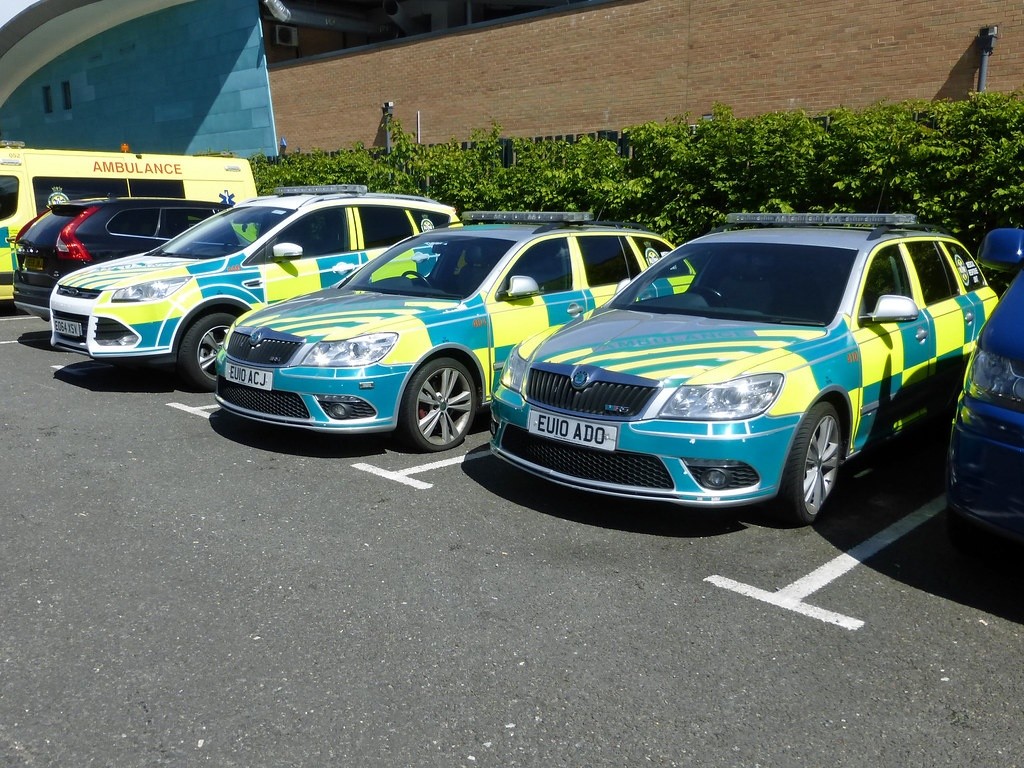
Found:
[10,195,297,330]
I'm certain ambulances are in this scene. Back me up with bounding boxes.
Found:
[0,136,260,316]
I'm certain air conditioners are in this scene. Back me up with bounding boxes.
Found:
[273,25,298,47]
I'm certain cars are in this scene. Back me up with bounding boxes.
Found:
[943,226,1024,563]
[44,182,468,395]
[210,209,700,455]
[489,213,1000,528]
